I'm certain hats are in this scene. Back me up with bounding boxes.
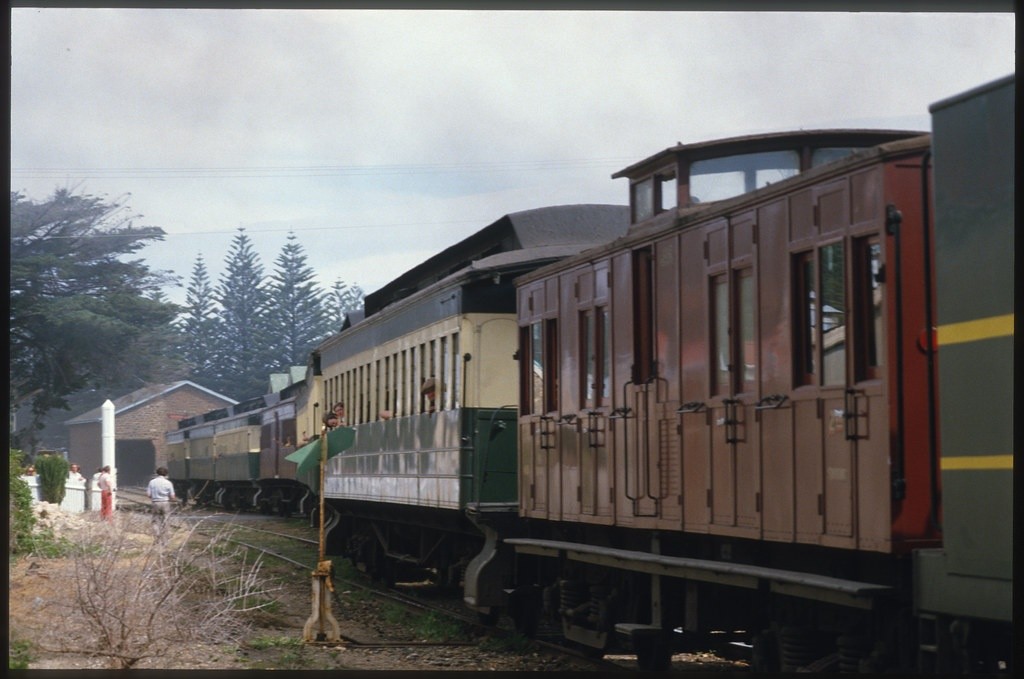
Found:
[420,378,435,394]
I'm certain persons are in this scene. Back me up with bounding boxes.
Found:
[422,377,436,412]
[93,465,113,521]
[147,468,176,536]
[334,402,347,426]
[285,436,290,446]
[68,464,84,480]
[321,412,337,430]
[25,466,38,476]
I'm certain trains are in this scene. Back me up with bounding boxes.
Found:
[167,68,1017,679]
